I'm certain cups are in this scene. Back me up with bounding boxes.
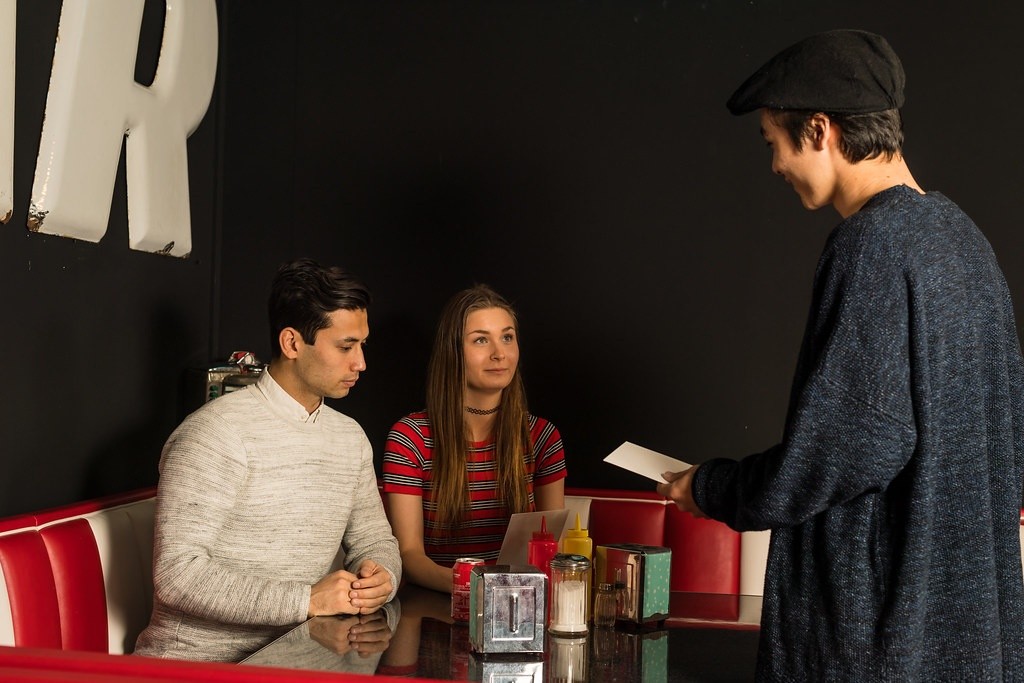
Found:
[547,554,589,638]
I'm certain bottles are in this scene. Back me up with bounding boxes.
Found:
[595,583,617,628]
[615,582,629,620]
[563,512,592,621]
[527,515,559,625]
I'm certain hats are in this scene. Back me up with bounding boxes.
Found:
[726,30,907,114]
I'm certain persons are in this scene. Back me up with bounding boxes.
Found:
[126,261,402,663]
[239,598,403,675]
[384,287,567,593]
[657,29,1024,683]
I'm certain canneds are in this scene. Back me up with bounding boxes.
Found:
[452,558,486,625]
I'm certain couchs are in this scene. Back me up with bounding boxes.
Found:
[0,482,772,682]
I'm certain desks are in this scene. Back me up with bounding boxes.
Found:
[234,582,762,682]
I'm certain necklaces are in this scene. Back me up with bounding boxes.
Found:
[464,403,503,415]
